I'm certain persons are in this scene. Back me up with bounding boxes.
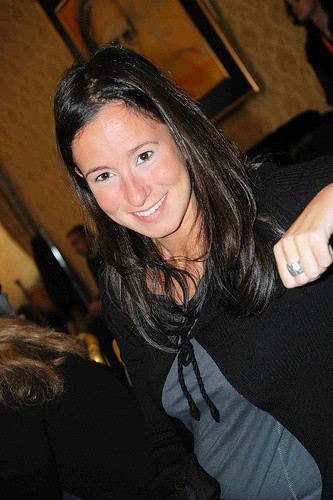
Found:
[52,46,333,500]
[78,2,217,99]
[1,315,184,500]
[285,0,333,110]
[68,226,132,376]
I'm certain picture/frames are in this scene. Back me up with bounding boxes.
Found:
[36,0,267,129]
